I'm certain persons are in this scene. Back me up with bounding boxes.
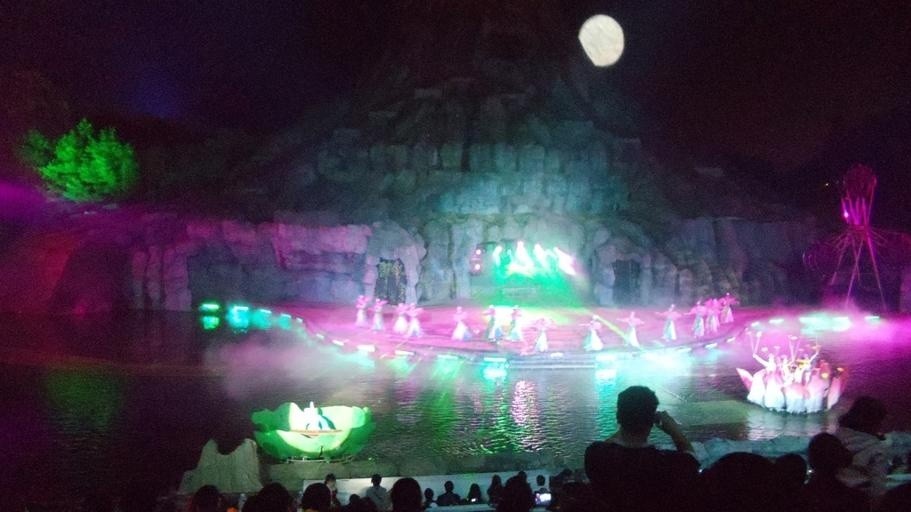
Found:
[532,316,551,352]
[718,290,740,324]
[449,305,472,342]
[575,314,603,353]
[734,335,848,416]
[389,300,409,335]
[508,303,526,342]
[652,303,683,343]
[370,297,385,331]
[613,309,646,348]
[194,467,583,510]
[352,295,368,328]
[683,298,707,339]
[304,400,321,430]
[698,432,910,510]
[583,381,698,512]
[832,394,891,512]
[480,304,502,343]
[703,296,722,334]
[402,302,424,339]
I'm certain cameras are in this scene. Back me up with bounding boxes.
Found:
[533,491,555,507]
[471,498,476,502]
